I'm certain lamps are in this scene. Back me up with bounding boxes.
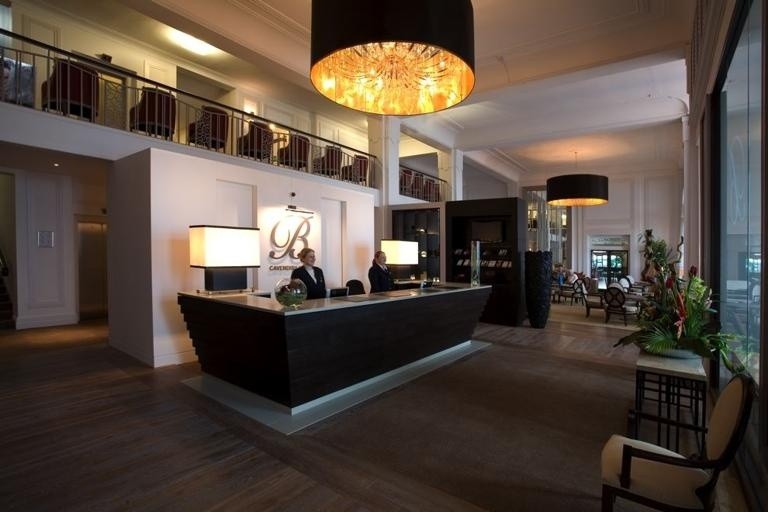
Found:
[309,0,475,116]
[546,152,609,208]
[380,239,419,279]
[188,225,261,293]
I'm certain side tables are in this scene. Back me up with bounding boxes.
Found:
[627,347,708,455]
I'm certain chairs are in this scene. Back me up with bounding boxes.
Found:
[40,57,101,123]
[127,85,176,140]
[240,121,273,162]
[346,279,366,296]
[277,134,440,202]
[601,372,757,512]
[551,272,654,327]
[188,104,228,151]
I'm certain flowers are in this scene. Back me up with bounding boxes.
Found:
[613,264,740,373]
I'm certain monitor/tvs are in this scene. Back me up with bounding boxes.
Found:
[330,288,347,297]
[424,282,432,287]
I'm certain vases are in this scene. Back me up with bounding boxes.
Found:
[635,342,702,360]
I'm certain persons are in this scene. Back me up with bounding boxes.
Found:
[557,267,567,285]
[289,248,326,299]
[566,269,579,285]
[368,251,395,292]
